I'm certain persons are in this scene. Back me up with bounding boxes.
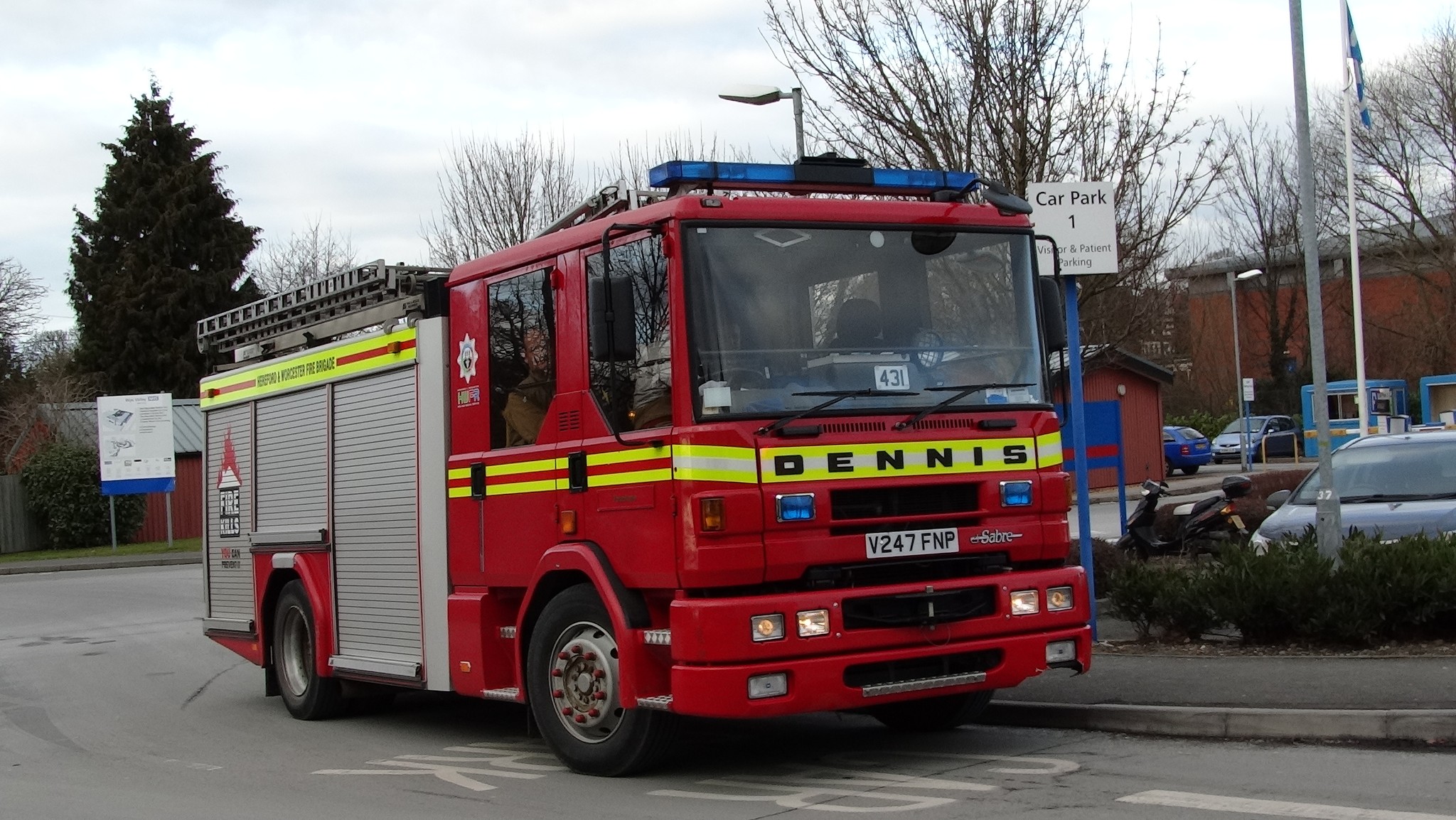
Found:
[505,326,554,446]
[631,305,749,428]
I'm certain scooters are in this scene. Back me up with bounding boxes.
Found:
[1113,475,1252,560]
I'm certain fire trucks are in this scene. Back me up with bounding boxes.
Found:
[195,152,1092,771]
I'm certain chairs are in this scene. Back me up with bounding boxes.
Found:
[828,299,885,356]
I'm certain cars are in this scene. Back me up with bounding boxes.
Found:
[1163,427,1212,477]
[1247,430,1456,558]
[1211,415,1305,465]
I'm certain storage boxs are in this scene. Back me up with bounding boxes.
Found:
[1222,475,1252,498]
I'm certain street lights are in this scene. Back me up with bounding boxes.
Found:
[1228,269,1263,472]
[718,87,806,198]
